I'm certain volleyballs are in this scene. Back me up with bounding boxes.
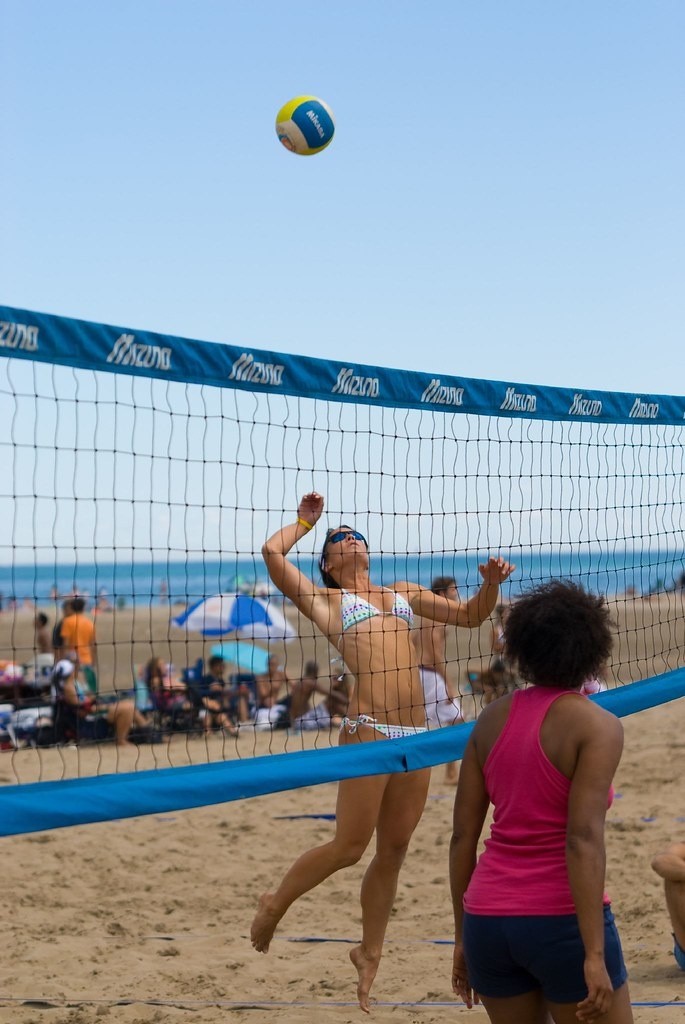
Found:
[273,94,340,156]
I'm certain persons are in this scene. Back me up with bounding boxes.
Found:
[1,573,684,791]
[650,842,684,967]
[448,580,634,1023]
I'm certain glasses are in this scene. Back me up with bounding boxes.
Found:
[325,531,368,546]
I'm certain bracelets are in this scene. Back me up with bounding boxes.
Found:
[297,517,313,530]
[251,491,517,1013]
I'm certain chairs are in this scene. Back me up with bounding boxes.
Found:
[51,698,125,747]
[146,678,206,732]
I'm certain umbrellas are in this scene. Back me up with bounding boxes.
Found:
[170,593,297,689]
